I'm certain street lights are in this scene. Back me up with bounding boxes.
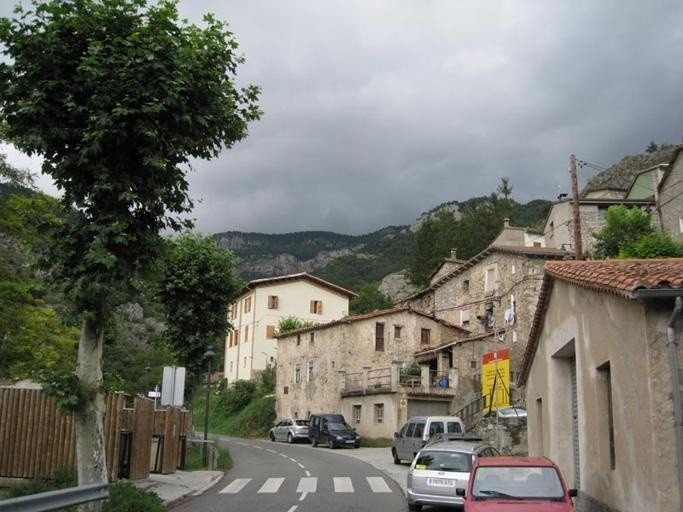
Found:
[202,344,218,466]
[145,364,153,398]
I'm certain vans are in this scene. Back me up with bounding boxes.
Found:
[391,415,464,465]
[308,413,361,450]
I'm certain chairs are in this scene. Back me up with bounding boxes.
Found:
[483,474,501,484]
[527,473,545,484]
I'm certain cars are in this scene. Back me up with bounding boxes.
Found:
[426,432,483,444]
[269,418,310,443]
[406,440,501,512]
[455,455,577,512]
